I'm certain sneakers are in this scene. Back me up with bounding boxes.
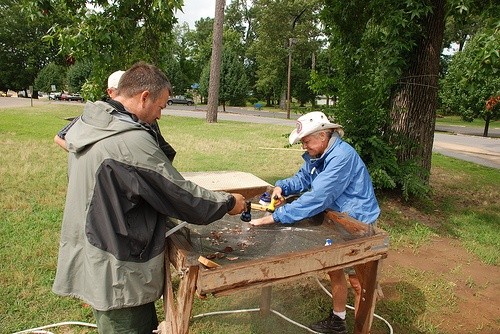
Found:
[310,309,347,334]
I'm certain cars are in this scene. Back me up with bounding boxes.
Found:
[16,89,84,101]
[166,95,195,106]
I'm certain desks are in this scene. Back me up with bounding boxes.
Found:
[165,186,389,334]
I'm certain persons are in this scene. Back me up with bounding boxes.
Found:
[52,61,249,333]
[55,69,126,154]
[248,110,381,334]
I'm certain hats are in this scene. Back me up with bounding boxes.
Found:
[288,111,343,145]
[108,71,127,89]
